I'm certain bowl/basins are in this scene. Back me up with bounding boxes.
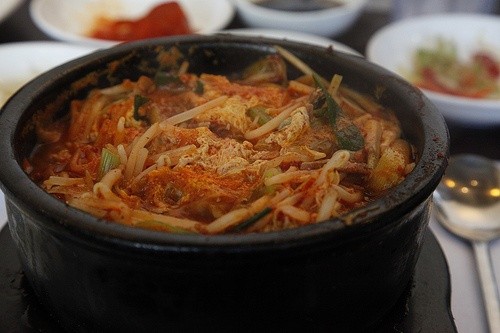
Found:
[230,0,367,40]
[0,33,452,333]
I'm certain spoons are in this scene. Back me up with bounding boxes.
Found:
[432,154,500,333]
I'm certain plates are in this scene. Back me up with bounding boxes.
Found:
[0,0,23,21]
[0,41,128,232]
[365,11,500,126]
[27,0,235,47]
[214,28,364,59]
